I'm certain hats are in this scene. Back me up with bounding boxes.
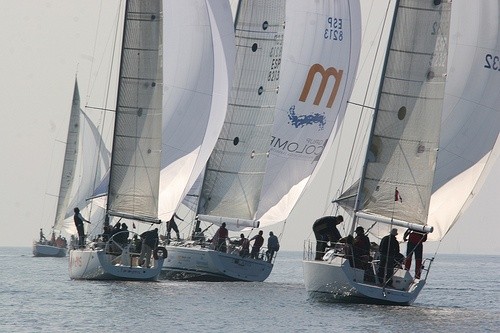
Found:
[354,227,364,232]
[390,228,398,235]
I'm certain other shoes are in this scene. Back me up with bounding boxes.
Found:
[315,257,323,261]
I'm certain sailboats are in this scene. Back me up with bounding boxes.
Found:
[303,0,500,307]
[32,71,112,259]
[68,0,363,281]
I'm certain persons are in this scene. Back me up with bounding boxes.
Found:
[250,230,264,259]
[138,228,159,267]
[236,234,250,257]
[102,223,129,241]
[313,215,343,261]
[379,229,400,288]
[404,228,427,280]
[74,207,91,248]
[39,228,47,242]
[58,235,68,246]
[167,213,184,240]
[217,222,231,252]
[266,231,278,263]
[340,226,370,280]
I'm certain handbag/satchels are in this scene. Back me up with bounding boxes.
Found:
[275,243,279,251]
